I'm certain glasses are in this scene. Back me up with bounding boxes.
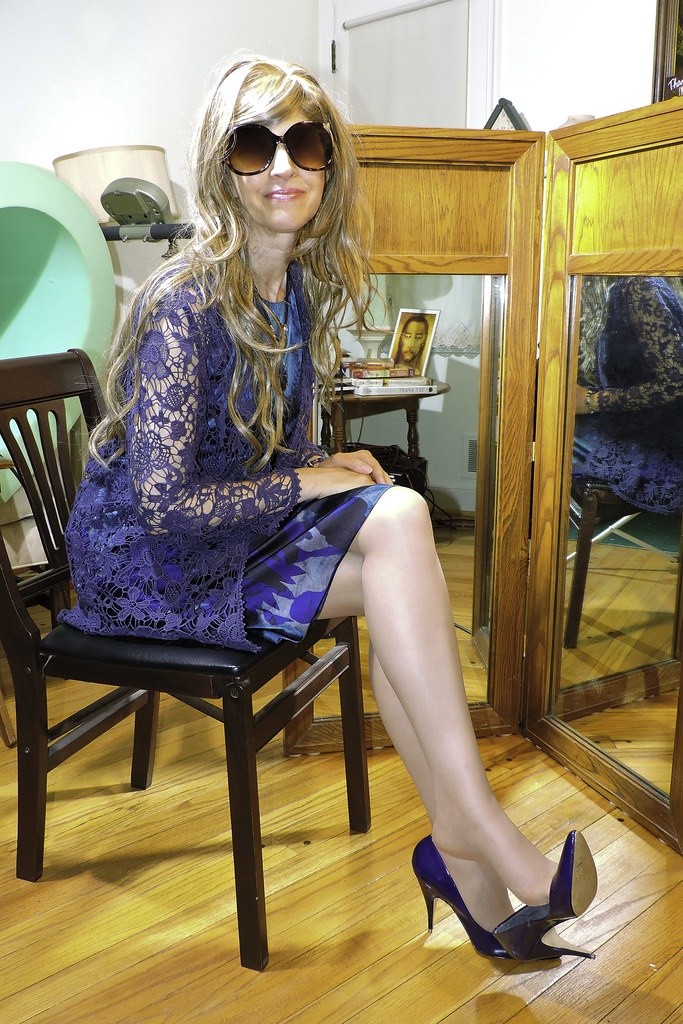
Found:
[220,121,334,176]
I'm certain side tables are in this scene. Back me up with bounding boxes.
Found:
[320,378,452,459]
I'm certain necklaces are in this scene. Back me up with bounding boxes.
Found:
[251,275,294,390]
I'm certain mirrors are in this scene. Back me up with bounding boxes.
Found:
[284,94,682,856]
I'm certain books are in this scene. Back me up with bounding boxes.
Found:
[351,378,433,386]
[354,386,438,396]
[346,368,414,378]
[341,355,395,369]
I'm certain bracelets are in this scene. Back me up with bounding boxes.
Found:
[307,457,327,469]
[586,389,596,415]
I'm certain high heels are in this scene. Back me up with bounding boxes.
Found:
[492,830,597,962]
[411,832,526,961]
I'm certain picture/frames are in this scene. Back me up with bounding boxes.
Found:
[388,307,443,377]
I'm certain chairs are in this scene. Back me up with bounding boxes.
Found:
[562,473,682,667]
[1,348,372,972]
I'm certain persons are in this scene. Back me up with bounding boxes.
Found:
[565,273,682,514]
[64,58,601,962]
